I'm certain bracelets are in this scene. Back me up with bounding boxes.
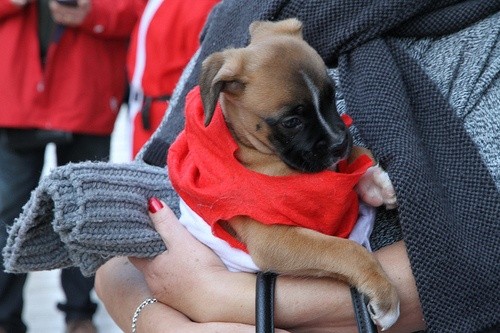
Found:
[131,297,158,332]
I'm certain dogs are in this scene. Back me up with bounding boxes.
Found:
[177,17,403,332]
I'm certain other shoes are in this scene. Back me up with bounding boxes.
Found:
[61,317,98,333]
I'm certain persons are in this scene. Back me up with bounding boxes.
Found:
[94,0,499,331]
[0,0,144,333]
[89,0,219,332]
